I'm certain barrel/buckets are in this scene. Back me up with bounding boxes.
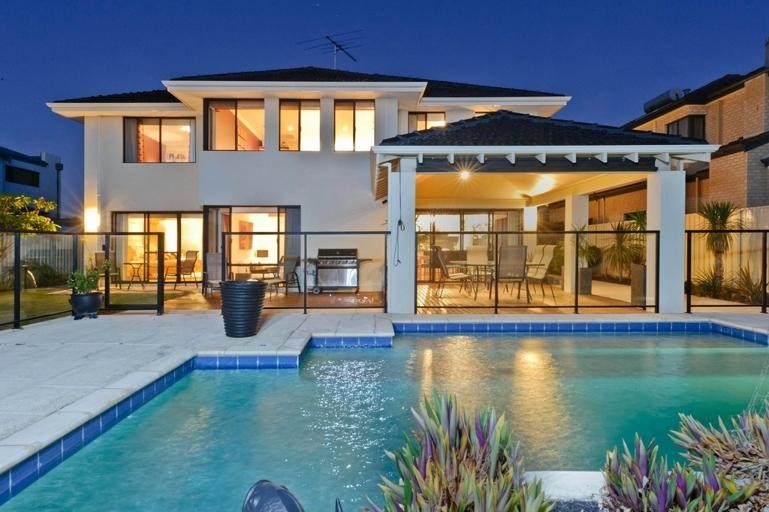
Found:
[218,279,268,337]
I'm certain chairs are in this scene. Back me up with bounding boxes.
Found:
[94,253,121,291]
[164,251,199,290]
[431,245,557,303]
[202,253,233,297]
[262,255,300,302]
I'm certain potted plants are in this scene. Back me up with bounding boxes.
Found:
[560,222,599,296]
[65,258,112,320]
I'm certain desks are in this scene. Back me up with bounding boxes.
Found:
[124,263,147,291]
